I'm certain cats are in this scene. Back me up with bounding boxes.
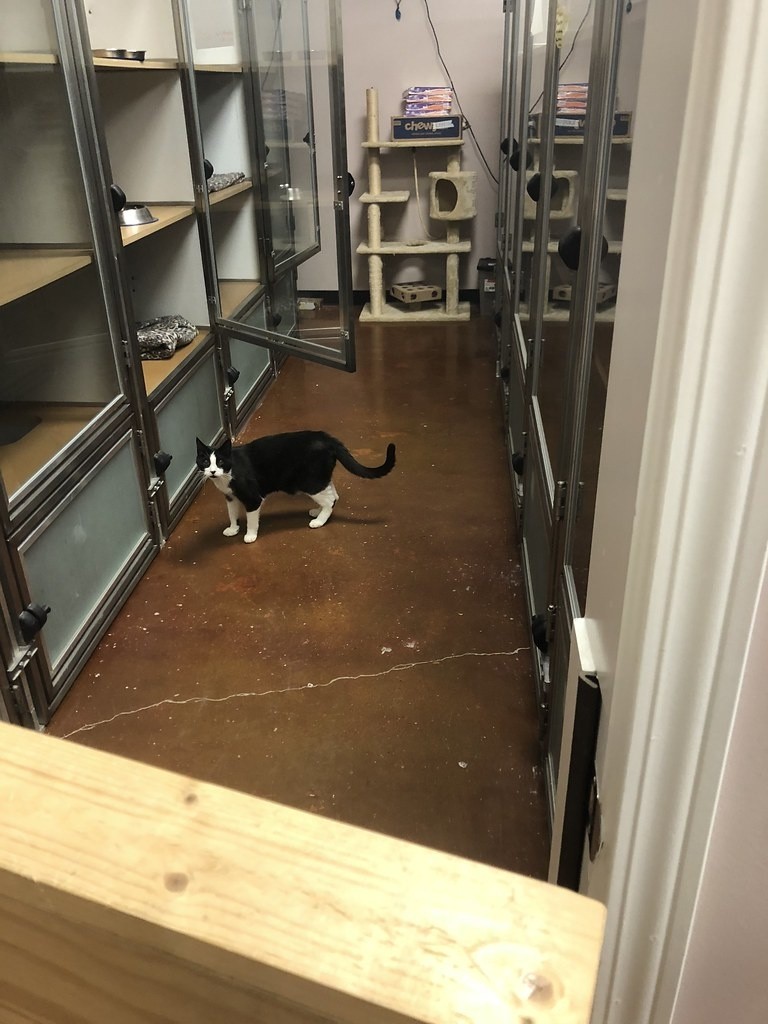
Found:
[195,429,397,544]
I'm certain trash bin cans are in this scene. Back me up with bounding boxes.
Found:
[477,257,498,316]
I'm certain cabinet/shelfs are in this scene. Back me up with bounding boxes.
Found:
[518,114,634,323]
[357,89,477,323]
[0,0,357,732]
[493,0,649,751]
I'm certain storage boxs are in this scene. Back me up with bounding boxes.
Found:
[553,114,586,139]
[390,114,463,143]
